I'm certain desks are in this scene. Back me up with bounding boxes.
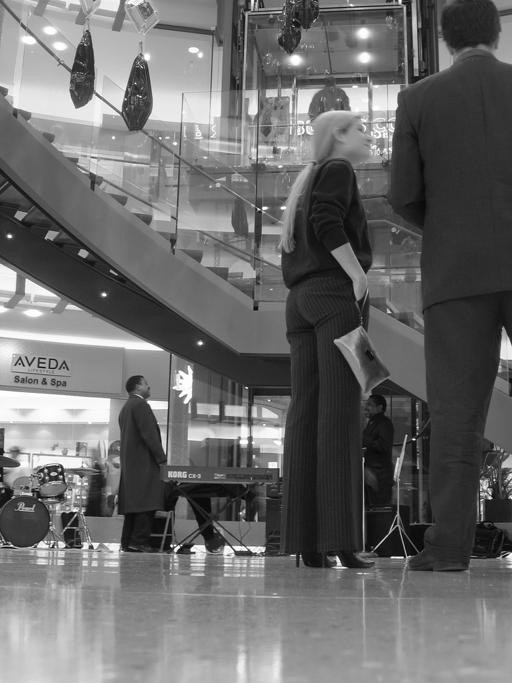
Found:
[170,479,262,557]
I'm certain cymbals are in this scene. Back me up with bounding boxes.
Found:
[63,467,100,478]
[0,456,21,468]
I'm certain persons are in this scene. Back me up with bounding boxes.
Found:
[386,0,512,574]
[4,445,32,497]
[307,75,352,122]
[274,108,380,569]
[359,392,396,508]
[117,373,170,552]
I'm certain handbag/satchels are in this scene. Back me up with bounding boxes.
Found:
[332,287,391,395]
[473,518,505,559]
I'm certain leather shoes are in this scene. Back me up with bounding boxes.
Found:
[121,543,157,553]
[407,548,470,572]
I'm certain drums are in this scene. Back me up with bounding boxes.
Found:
[0,495,51,548]
[34,462,68,498]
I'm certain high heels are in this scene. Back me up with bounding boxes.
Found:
[295,551,337,568]
[322,548,375,569]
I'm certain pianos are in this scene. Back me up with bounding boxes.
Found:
[160,465,279,483]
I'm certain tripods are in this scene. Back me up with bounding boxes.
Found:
[368,476,421,559]
[34,474,97,550]
